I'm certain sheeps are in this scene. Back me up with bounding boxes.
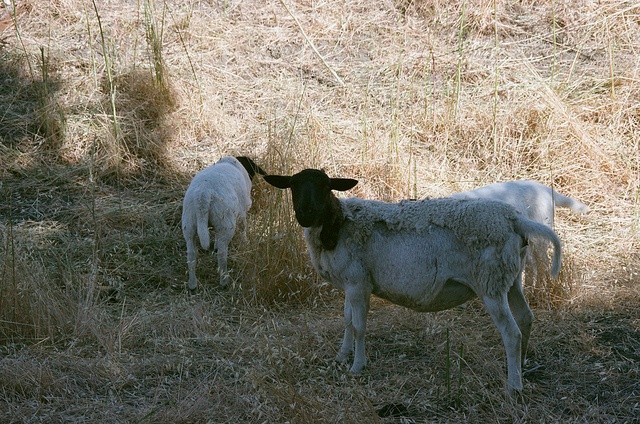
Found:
[261,166,568,404]
[180,151,269,292]
[422,180,591,306]
